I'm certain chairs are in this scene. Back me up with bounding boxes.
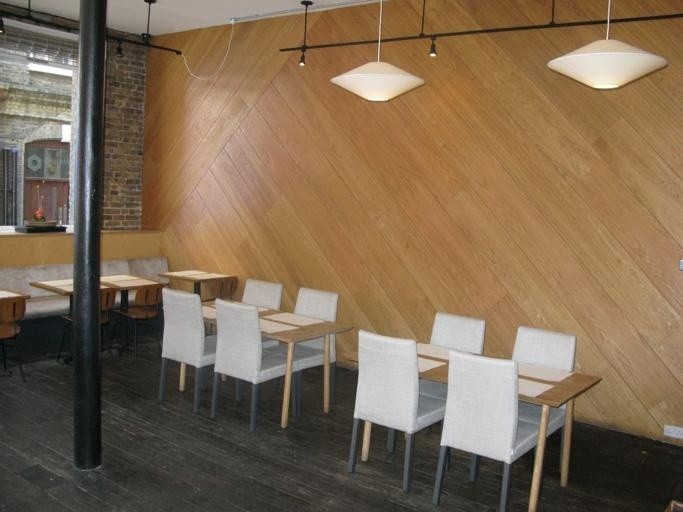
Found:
[345,310,576,512]
[0,273,340,430]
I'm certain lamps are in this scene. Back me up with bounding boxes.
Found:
[429,36,439,58]
[330,0,427,103]
[545,2,669,91]
[297,45,308,67]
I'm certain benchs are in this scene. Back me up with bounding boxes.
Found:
[1,254,169,355]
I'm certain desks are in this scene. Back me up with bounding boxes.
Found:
[337,338,603,512]
